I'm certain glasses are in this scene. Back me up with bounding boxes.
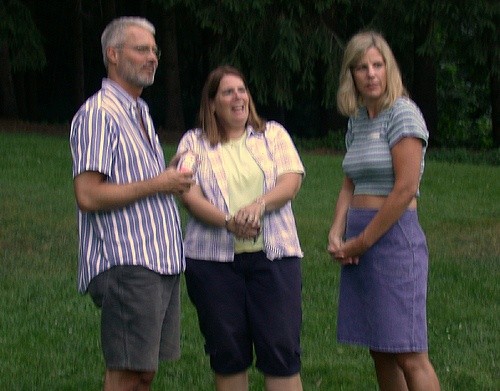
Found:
[112,45,161,60]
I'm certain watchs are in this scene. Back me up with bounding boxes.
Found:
[225,214,232,231]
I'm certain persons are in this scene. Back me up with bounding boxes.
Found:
[328,31,441,391]
[176,66,305,391]
[70,17,199,391]
